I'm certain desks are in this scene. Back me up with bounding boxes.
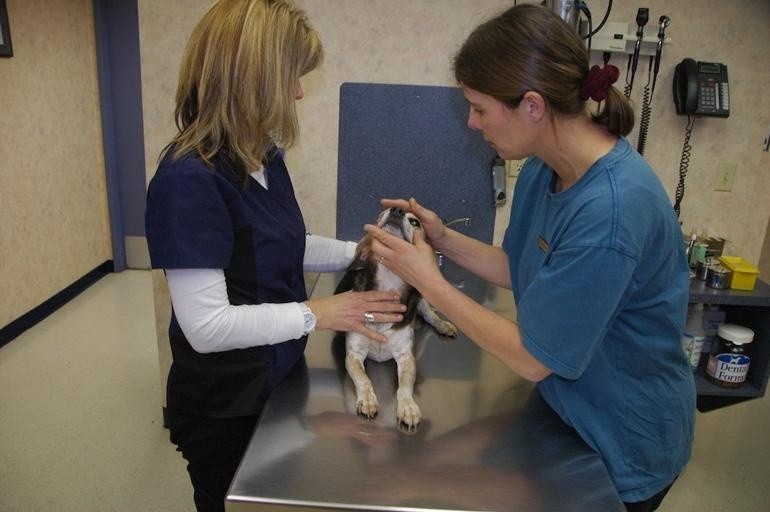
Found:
[222,263,628,510]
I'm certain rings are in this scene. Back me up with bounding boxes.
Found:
[379,255,384,264]
[364,312,376,324]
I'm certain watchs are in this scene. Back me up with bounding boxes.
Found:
[298,300,318,336]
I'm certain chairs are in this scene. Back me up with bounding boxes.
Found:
[335,81,500,252]
[413,264,493,379]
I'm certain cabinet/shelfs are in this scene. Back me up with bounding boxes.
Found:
[684,269,770,413]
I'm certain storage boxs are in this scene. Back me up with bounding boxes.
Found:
[720,255,760,292]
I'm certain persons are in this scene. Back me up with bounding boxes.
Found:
[358,1,701,512]
[143,2,408,512]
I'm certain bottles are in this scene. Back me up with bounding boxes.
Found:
[708,324,756,388]
[704,265,732,289]
[681,301,707,376]
[695,256,721,281]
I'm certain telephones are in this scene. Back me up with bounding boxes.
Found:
[674,58,731,117]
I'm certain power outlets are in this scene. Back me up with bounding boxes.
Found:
[508,157,526,178]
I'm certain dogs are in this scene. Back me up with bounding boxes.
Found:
[330,206,458,433]
